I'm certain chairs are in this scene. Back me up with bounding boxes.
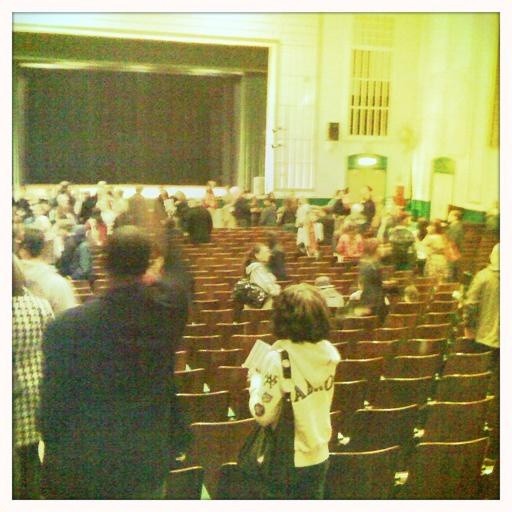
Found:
[70,220,499,501]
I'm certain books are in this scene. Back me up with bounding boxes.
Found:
[238,339,272,370]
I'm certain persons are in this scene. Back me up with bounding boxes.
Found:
[297,182,467,321]
[242,230,286,310]
[13,262,57,499]
[245,279,346,499]
[30,221,193,499]
[482,200,499,234]
[15,179,213,280]
[202,180,303,229]
[460,240,499,363]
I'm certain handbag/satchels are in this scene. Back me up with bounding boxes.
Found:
[237,345,300,498]
[231,276,269,309]
